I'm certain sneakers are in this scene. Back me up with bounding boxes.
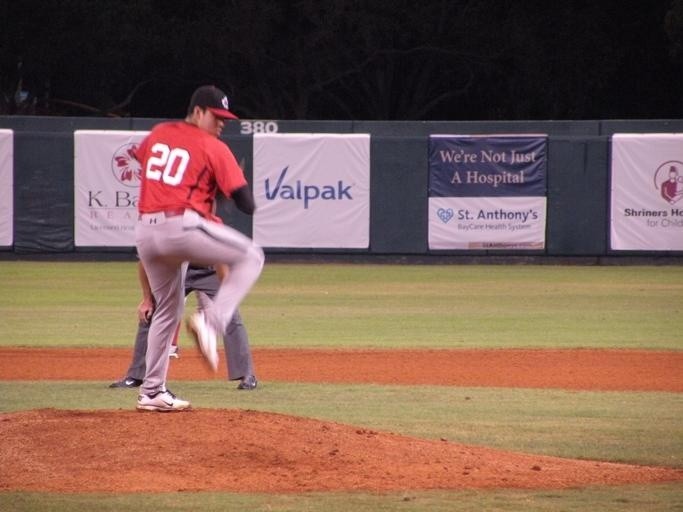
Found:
[187,312,221,374]
[136,389,191,413]
[237,375,259,390]
[108,376,143,390]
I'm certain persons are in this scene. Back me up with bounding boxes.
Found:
[108,192,259,392]
[131,85,267,415]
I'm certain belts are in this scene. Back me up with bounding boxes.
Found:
[137,209,185,221]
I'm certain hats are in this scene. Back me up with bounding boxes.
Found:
[186,85,241,121]
